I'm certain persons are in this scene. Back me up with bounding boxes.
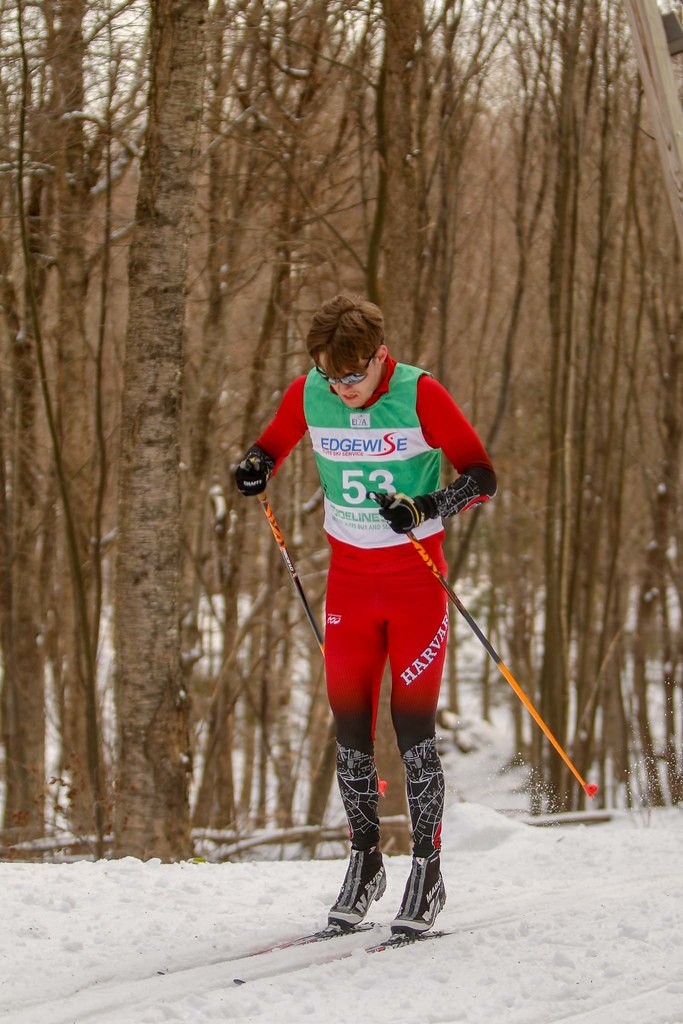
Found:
[236,296,498,939]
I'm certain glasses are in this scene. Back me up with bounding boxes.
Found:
[316,351,377,385]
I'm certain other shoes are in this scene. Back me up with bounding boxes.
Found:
[391,856,446,934]
[328,846,388,928]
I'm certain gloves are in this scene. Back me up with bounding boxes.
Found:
[235,444,274,496]
[377,494,438,534]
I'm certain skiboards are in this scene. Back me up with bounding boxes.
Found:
[154,919,452,985]
